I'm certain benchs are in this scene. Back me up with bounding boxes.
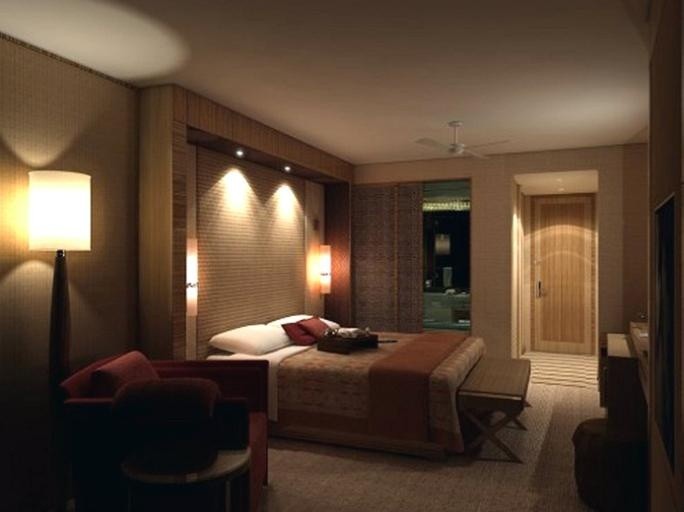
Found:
[457,353,532,467]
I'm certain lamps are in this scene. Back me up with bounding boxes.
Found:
[434,234,452,292]
[319,244,332,294]
[185,237,199,317]
[26,169,92,512]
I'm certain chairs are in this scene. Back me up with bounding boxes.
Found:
[59,353,270,511]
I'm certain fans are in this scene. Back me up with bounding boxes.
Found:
[414,121,510,160]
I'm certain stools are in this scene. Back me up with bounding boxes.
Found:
[123,441,252,511]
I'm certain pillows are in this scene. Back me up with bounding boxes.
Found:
[208,312,340,356]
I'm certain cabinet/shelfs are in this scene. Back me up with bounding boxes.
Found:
[607,333,639,451]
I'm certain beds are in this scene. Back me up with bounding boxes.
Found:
[207,315,485,462]
